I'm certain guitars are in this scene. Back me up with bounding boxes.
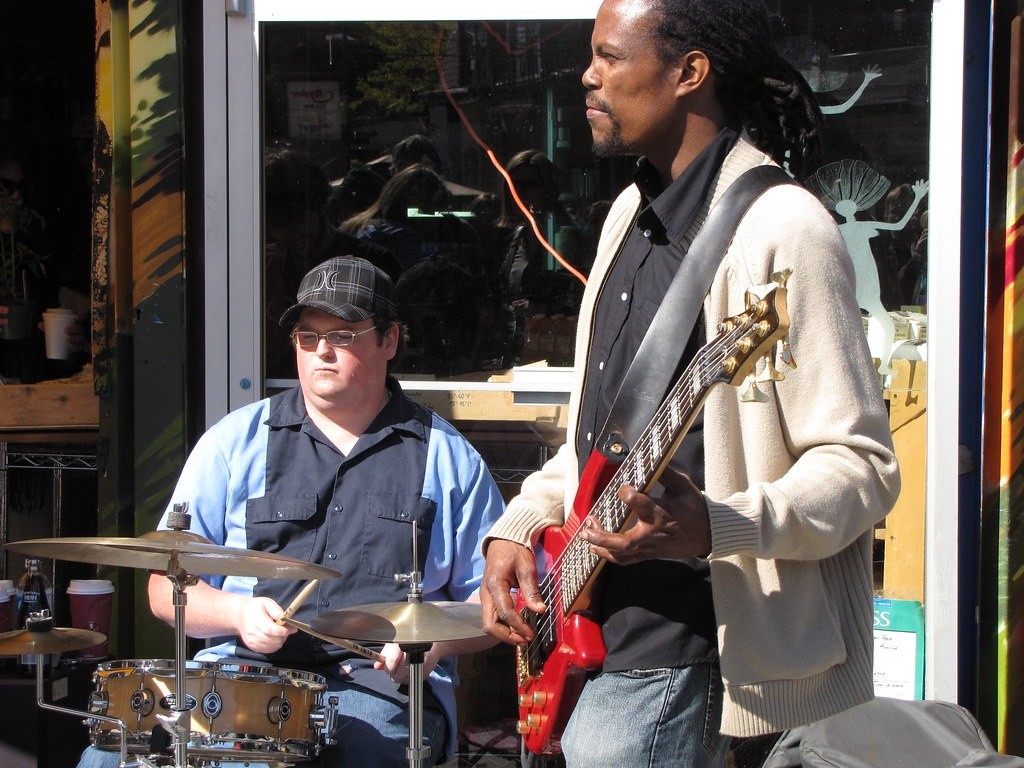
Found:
[507,268,791,756]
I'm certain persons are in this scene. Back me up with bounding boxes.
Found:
[259,134,929,380]
[480,0,901,768]
[74,254,507,768]
[0,151,92,515]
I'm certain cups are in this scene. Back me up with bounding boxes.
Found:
[0,300,30,340]
[42,308,78,359]
[0,579,17,634]
[66,580,116,660]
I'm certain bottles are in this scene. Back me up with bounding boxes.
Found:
[15,559,53,679]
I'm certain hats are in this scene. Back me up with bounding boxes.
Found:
[279,255,397,328]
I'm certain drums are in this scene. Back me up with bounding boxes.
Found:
[87,660,329,761]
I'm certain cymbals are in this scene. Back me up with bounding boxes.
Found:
[284,597,499,642]
[0,530,345,581]
[0,627,109,658]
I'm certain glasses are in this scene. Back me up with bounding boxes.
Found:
[289,326,378,347]
